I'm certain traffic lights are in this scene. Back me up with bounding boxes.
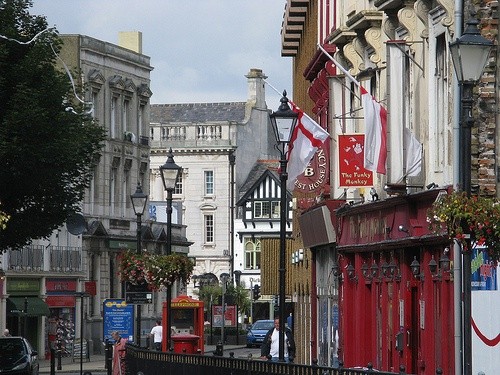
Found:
[254,284,261,300]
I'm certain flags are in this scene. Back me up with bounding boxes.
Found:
[360,86,388,176]
[277,101,330,191]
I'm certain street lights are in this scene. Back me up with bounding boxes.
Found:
[159,146,183,354]
[129,181,151,349]
[447,8,497,375]
[267,88,301,363]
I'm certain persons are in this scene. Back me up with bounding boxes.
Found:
[261,318,297,364]
[1,329,12,337]
[112,331,123,345]
[148,321,163,351]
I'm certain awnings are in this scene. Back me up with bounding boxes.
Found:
[7,296,50,317]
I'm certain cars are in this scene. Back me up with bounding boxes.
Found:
[246,320,275,348]
[0,336,39,375]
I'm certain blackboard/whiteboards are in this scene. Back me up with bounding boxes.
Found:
[72,337,90,360]
